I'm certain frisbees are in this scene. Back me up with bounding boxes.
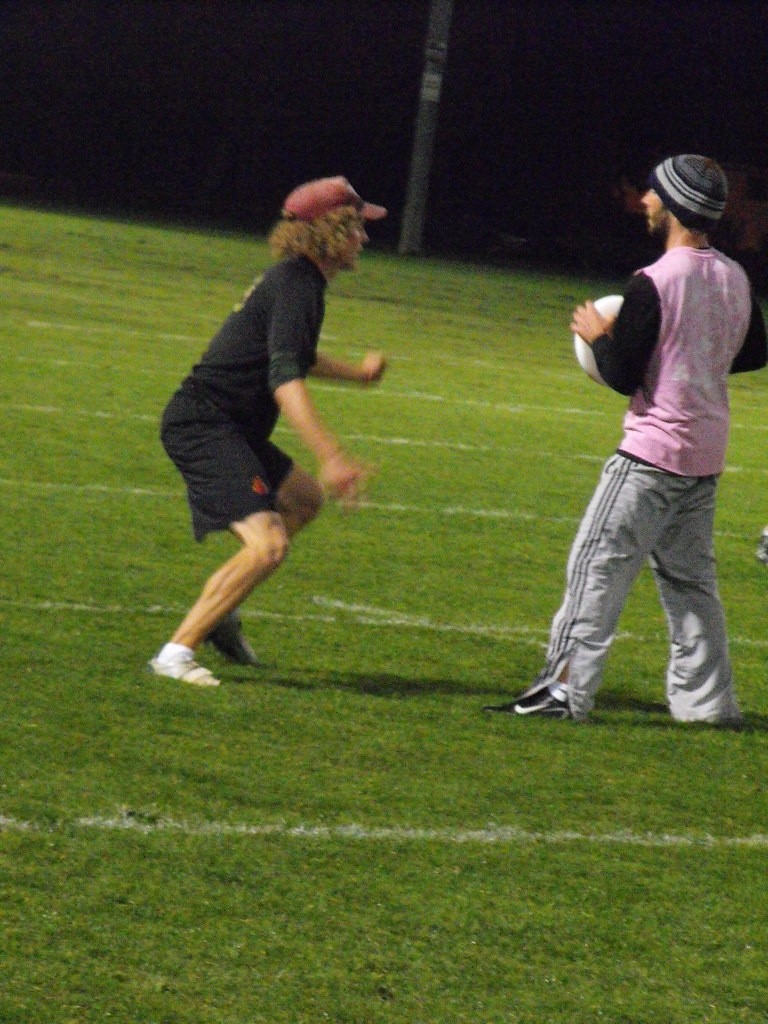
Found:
[576,295,631,386]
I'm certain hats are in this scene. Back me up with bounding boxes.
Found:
[651,154,726,228]
[282,175,387,221]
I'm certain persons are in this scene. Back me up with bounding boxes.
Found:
[481,155,768,725]
[149,174,387,687]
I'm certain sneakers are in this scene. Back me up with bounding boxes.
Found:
[482,679,590,717]
[152,659,222,686]
[209,609,259,667]
